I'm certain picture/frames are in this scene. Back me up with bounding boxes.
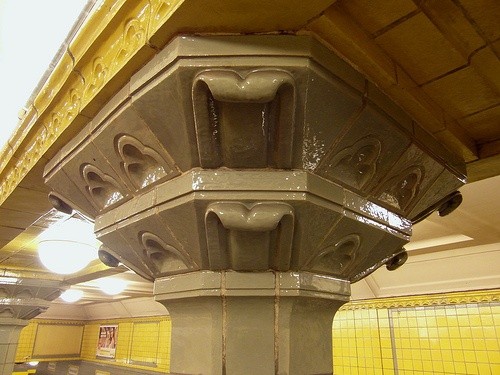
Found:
[95,322,120,362]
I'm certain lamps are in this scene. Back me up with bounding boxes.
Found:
[37,239,98,276]
[60,289,84,303]
[99,278,130,296]
